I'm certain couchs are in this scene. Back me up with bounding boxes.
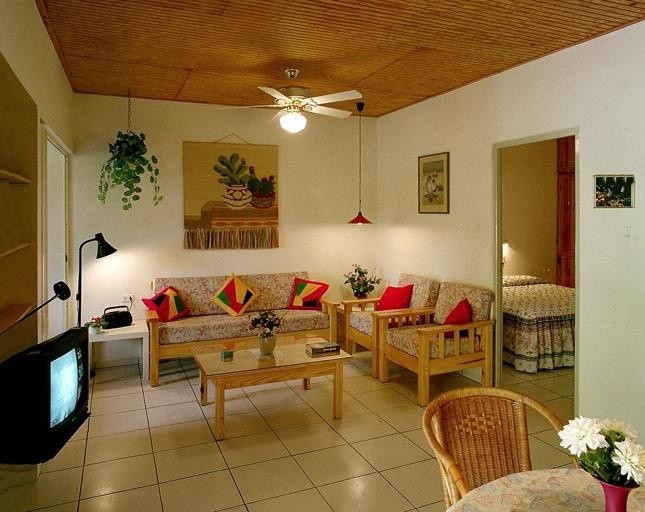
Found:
[146,272,340,388]
[343,266,438,376]
[380,282,496,406]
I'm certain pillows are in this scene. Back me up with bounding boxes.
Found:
[209,273,257,315]
[503,275,542,286]
[443,300,471,337]
[140,288,189,321]
[379,284,412,309]
[288,277,328,311]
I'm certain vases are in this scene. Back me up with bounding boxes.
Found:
[597,486,633,510]
[256,335,278,355]
[252,197,276,209]
[356,296,370,306]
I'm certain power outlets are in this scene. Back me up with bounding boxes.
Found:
[119,294,133,302]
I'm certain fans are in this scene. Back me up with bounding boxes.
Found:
[215,67,362,134]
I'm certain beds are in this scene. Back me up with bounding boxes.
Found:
[503,282,574,374]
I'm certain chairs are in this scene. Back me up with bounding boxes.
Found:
[422,386,579,506]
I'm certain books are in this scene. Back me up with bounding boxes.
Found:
[304,341,342,355]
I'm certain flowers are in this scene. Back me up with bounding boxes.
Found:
[344,262,380,295]
[557,415,645,484]
[251,165,276,194]
[250,312,284,337]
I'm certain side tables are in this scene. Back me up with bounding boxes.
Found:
[87,320,149,386]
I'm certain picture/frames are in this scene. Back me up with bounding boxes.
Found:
[593,173,636,210]
[417,152,449,216]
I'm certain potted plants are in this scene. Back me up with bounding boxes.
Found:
[214,154,251,210]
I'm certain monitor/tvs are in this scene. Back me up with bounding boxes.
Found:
[0,325,89,464]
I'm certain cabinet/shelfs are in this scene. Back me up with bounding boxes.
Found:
[0,52,43,355]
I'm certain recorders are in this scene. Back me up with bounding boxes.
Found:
[100,305,132,329]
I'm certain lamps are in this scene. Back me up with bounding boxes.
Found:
[76,232,117,325]
[20,279,71,325]
[274,108,309,133]
[346,101,374,228]
